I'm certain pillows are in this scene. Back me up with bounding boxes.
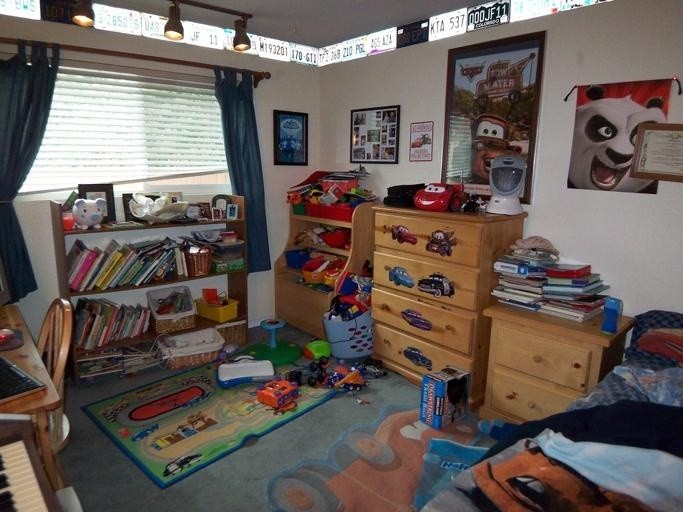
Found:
[624,311,683,370]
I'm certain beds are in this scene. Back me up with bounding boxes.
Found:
[418,362,683,512]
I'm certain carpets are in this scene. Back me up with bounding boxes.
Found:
[81,341,339,489]
[266,405,488,512]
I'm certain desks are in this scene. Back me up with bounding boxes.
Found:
[0,304,71,491]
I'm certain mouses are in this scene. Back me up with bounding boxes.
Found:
[0,329,13,342]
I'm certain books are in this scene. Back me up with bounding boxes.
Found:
[490,252,613,329]
[65,235,189,379]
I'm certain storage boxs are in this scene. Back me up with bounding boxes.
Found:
[194,295,240,324]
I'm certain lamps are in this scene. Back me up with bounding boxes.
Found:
[163,0,253,52]
[69,0,96,28]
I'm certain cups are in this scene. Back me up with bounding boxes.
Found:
[601,294,623,333]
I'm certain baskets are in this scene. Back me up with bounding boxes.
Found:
[323,309,374,359]
[146,285,196,334]
[218,320,247,346]
[183,240,211,276]
[167,350,218,369]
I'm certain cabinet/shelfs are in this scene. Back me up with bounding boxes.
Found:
[274,200,382,340]
[368,205,528,412]
[49,193,248,389]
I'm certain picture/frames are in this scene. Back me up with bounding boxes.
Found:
[441,30,546,204]
[78,183,116,222]
[351,105,399,165]
[409,121,434,163]
[629,123,683,183]
[273,108,308,167]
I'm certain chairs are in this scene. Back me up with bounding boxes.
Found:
[36,297,72,487]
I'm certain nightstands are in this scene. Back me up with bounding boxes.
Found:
[479,304,633,425]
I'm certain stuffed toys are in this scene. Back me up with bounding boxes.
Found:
[71,197,107,231]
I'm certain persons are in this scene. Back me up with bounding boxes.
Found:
[475,398,683,460]
